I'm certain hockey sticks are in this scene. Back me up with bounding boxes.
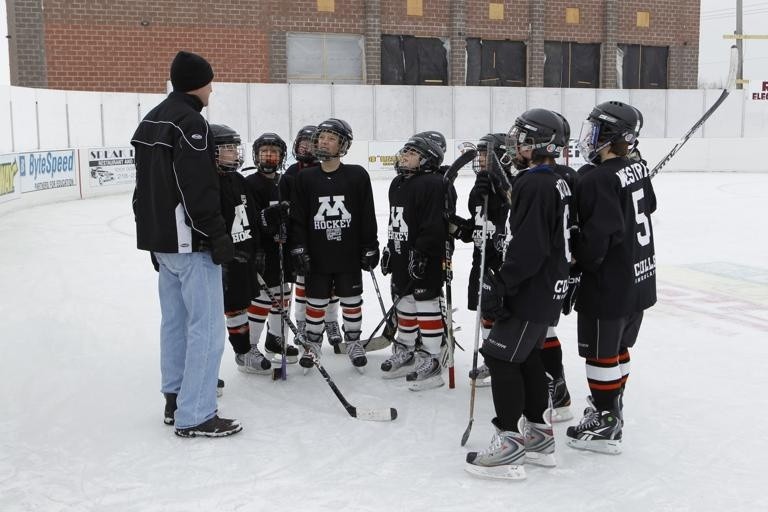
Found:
[334,309,396,355]
[443,150,478,388]
[461,192,488,445]
[647,44,738,181]
[256,271,397,421]
[271,200,286,381]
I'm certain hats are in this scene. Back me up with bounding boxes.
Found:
[170,51,215,92]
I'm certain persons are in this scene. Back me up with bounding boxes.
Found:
[465,106,573,480]
[205,106,657,423]
[288,117,380,374]
[128,49,245,440]
[558,100,658,454]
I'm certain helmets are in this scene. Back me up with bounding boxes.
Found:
[577,101,644,167]
[252,132,287,174]
[471,133,517,175]
[504,108,571,170]
[292,125,320,164]
[393,137,444,179]
[209,123,246,173]
[408,131,447,153]
[311,118,353,163]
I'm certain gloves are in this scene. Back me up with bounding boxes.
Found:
[449,214,467,240]
[257,201,294,228]
[408,249,430,282]
[561,262,582,316]
[249,248,266,274]
[481,275,504,322]
[380,247,391,276]
[210,234,235,266]
[359,241,380,271]
[293,253,311,278]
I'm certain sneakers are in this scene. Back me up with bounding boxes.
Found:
[466,416,526,467]
[293,319,306,345]
[405,349,442,381]
[173,414,244,439]
[547,376,571,409]
[325,321,343,346]
[298,330,323,368]
[469,364,491,380]
[583,395,624,428]
[234,351,272,370]
[163,404,219,426]
[217,378,224,388]
[380,343,415,373]
[265,332,299,356]
[341,324,368,367]
[249,344,264,357]
[524,421,556,454]
[566,410,623,440]
